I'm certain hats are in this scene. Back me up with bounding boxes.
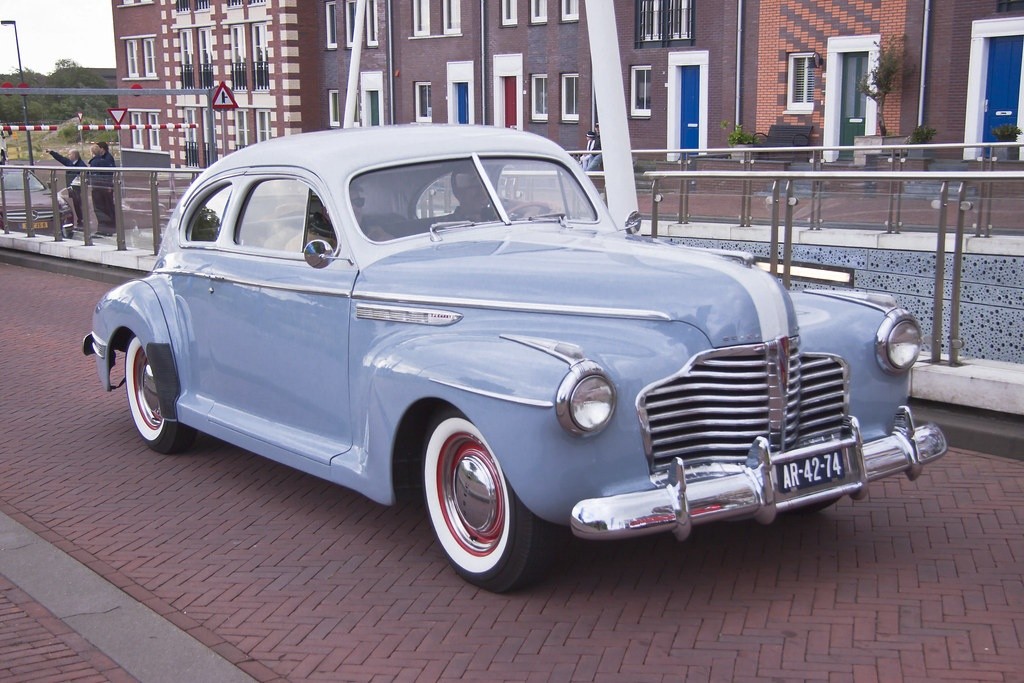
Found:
[96,142,108,151]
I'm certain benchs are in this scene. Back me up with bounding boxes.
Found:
[752,125,813,162]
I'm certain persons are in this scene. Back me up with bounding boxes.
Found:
[284,185,397,253]
[450,165,551,221]
[45,141,117,238]
[580,125,602,171]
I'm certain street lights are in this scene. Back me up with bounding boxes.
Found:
[1,20,35,166]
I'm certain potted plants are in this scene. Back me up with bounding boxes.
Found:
[720,120,762,159]
[991,123,1023,160]
[853,33,916,166]
[908,125,936,159]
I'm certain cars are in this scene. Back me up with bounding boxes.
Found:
[0,169,75,239]
[79,123,949,595]
